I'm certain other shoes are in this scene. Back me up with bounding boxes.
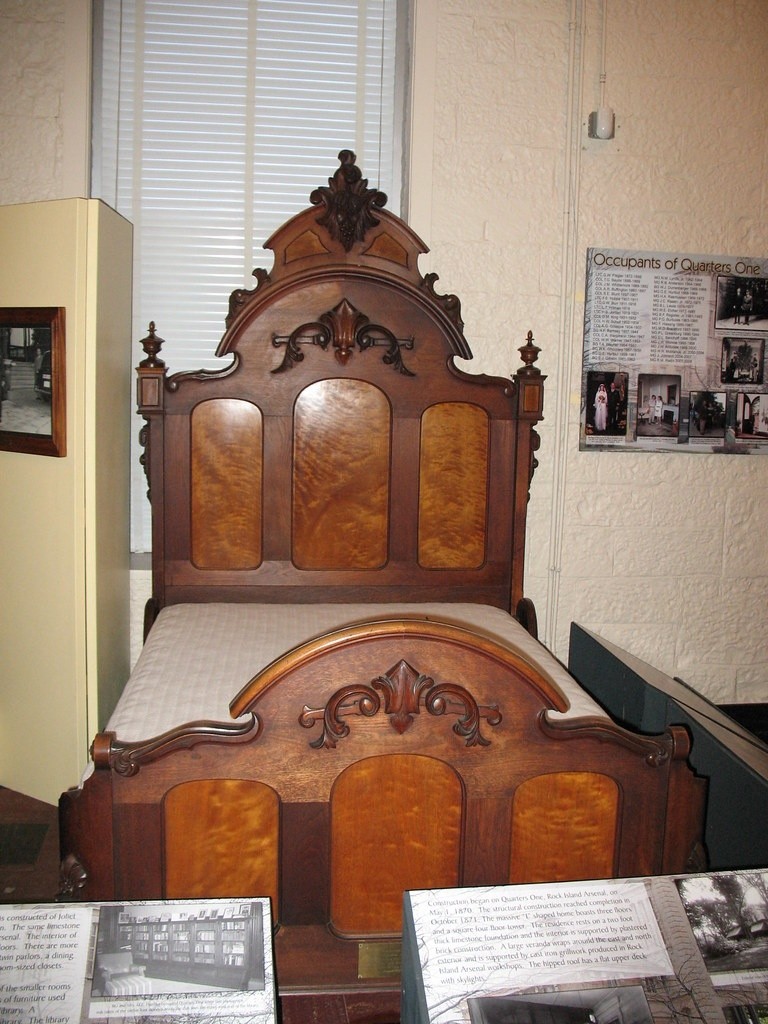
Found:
[652,422,655,424]
[660,423,661,426]
[656,422,658,424]
[648,423,651,425]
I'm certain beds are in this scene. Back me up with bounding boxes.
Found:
[55,149,710,998]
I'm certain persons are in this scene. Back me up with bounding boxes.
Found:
[699,400,722,435]
[595,383,620,430]
[732,286,752,325]
[648,395,662,427]
[725,357,758,383]
[34,348,44,400]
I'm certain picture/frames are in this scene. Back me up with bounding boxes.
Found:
[0,307,68,459]
